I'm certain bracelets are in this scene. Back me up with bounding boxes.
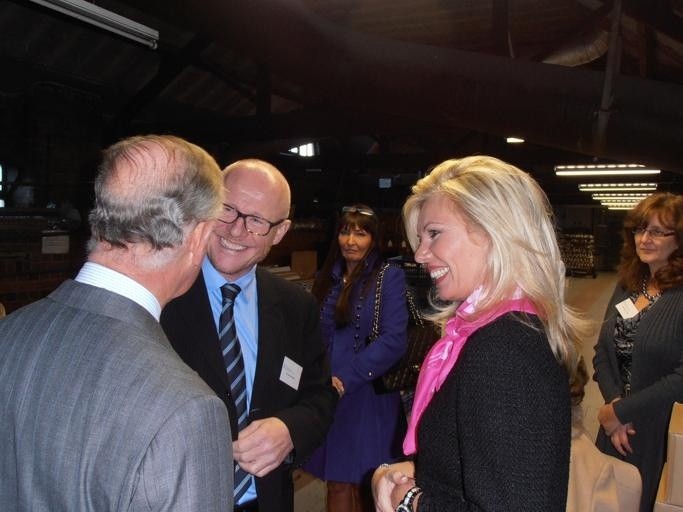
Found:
[378,460,392,472]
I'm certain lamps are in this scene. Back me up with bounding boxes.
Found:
[32,0,159,49]
[552,164,661,211]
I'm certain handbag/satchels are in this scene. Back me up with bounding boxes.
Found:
[367,260,446,397]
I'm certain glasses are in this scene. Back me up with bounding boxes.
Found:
[631,227,677,240]
[341,203,376,222]
[207,190,284,237]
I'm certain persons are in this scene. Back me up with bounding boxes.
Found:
[159,158,336,512]
[0,132,233,512]
[296,203,413,511]
[590,189,682,511]
[369,150,599,511]
[565,350,643,511]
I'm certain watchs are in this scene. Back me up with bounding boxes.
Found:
[395,485,422,511]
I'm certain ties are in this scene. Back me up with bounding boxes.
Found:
[212,280,255,497]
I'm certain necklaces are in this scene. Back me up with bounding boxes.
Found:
[641,269,664,302]
